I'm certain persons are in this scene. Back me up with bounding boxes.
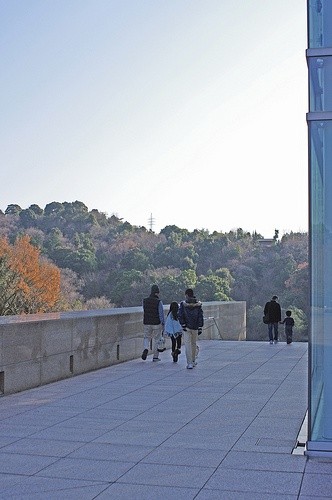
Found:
[179,288,204,369]
[164,302,184,362]
[264,296,281,344]
[280,310,294,344]
[142,284,164,360]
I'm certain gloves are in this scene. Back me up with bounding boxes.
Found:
[198,328,202,335]
[182,326,187,331]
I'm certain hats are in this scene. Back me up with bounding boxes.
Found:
[151,284,159,293]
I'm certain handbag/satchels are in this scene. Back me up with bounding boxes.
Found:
[263,313,269,324]
[157,331,166,352]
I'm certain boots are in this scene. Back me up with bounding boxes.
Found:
[174,349,179,358]
[171,352,176,362]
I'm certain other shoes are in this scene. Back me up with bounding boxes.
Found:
[187,366,192,369]
[274,340,277,343]
[270,340,273,344]
[142,348,148,360]
[153,358,161,362]
[193,362,196,366]
